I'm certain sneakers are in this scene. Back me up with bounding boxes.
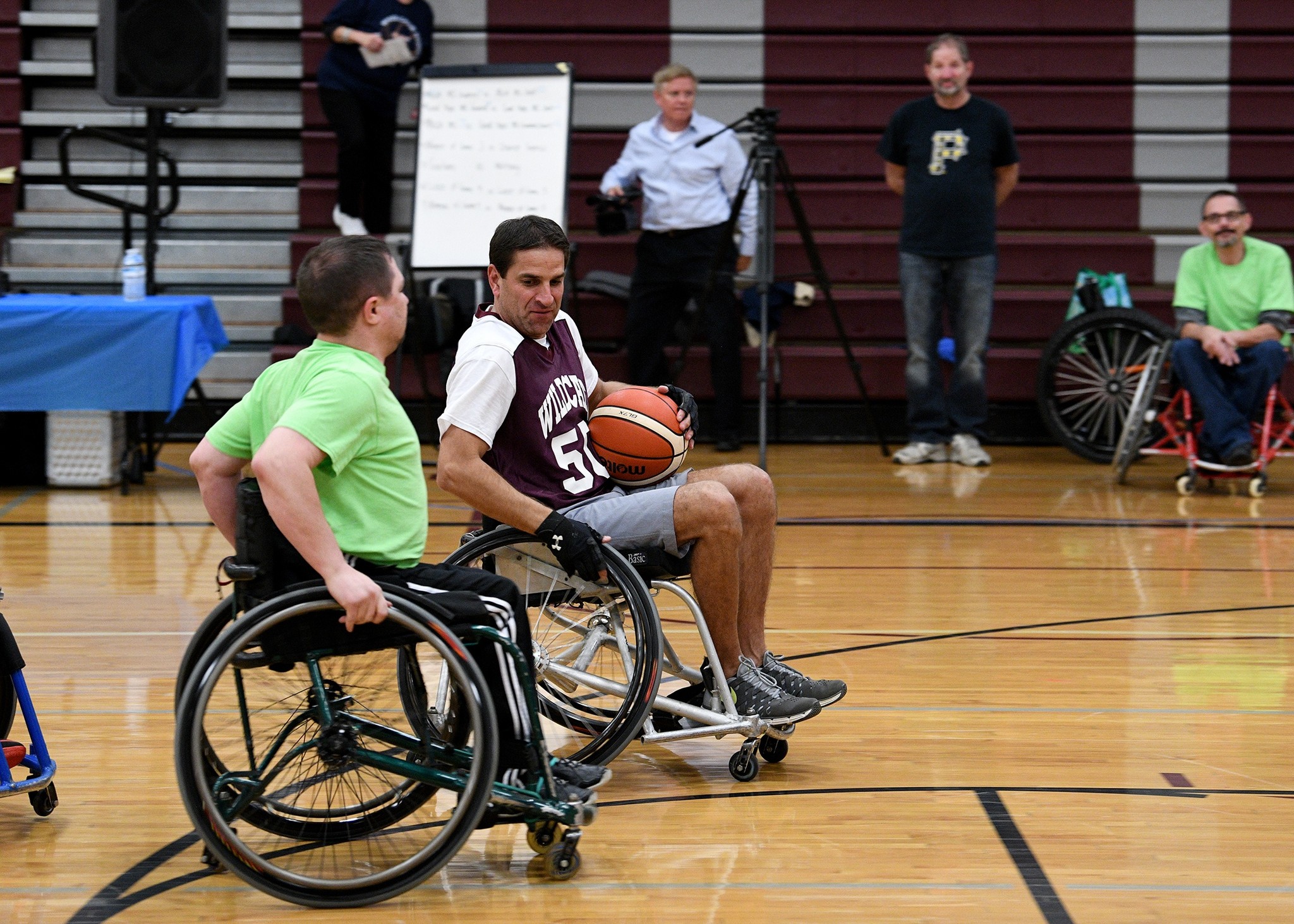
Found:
[760,650,847,708]
[519,742,614,790]
[951,433,992,468]
[701,655,822,729]
[486,760,599,820]
[891,440,949,466]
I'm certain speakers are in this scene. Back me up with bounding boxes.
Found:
[99,0,228,107]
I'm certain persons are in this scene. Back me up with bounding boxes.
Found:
[600,64,759,452]
[881,35,1022,467]
[319,0,434,235]
[437,216,848,726]
[189,236,613,809]
[1172,190,1294,466]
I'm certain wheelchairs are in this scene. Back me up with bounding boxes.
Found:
[1110,338,1294,499]
[397,446,795,782]
[173,480,596,909]
[0,589,59,816]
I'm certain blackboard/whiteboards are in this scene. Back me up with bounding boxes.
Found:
[403,58,577,281]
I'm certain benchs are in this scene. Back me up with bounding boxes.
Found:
[0,1,1294,427]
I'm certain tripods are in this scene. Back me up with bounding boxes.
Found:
[693,104,891,472]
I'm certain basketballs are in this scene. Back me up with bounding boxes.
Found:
[588,386,689,489]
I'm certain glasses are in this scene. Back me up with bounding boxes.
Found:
[1202,209,1249,222]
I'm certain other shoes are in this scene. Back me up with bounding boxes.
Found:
[1196,444,1255,477]
[333,204,369,238]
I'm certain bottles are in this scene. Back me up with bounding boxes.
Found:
[123,249,144,302]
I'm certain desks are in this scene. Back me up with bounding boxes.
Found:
[0,293,226,489]
